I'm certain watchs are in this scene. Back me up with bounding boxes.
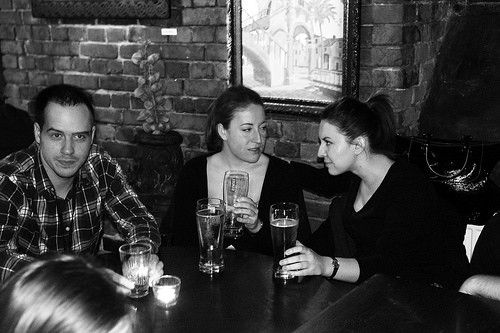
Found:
[327,257,340,280]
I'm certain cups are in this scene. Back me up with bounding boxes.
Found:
[196,209,225,274]
[197,197,226,214]
[119,243,152,298]
[152,275,181,309]
[270,203,299,279]
[223,170,249,236]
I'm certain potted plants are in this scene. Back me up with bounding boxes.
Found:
[130,40,184,190]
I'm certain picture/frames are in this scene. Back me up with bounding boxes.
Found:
[224,0,361,116]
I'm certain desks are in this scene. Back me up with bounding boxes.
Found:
[106,244,500,333]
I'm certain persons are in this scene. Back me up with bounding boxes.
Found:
[0,84,163,298]
[422,150,500,304]
[157,85,311,258]
[278,94,432,283]
[0,254,153,333]
